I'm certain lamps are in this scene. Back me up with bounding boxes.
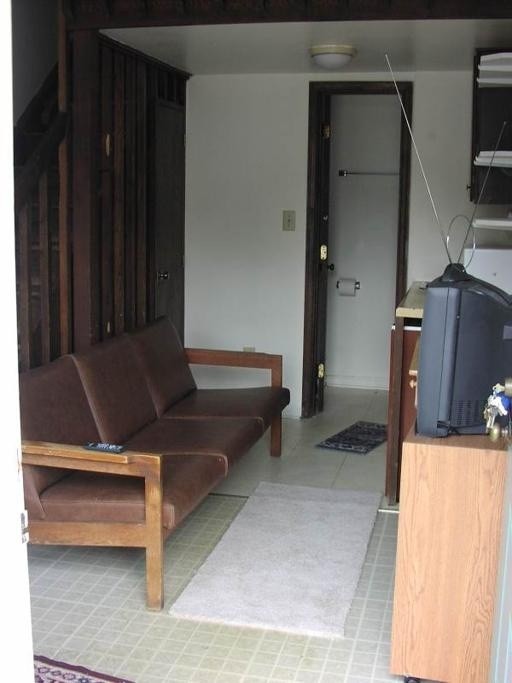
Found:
[307,45,359,70]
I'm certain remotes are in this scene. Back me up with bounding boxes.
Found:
[82,440,128,453]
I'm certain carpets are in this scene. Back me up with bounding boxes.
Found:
[315,420,388,455]
[169,479,383,642]
[33,656,136,683]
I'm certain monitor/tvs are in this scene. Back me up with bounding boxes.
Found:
[417,275,512,437]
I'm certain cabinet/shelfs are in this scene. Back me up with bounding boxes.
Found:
[149,100,186,342]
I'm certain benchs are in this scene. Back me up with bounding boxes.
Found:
[18,315,290,612]
